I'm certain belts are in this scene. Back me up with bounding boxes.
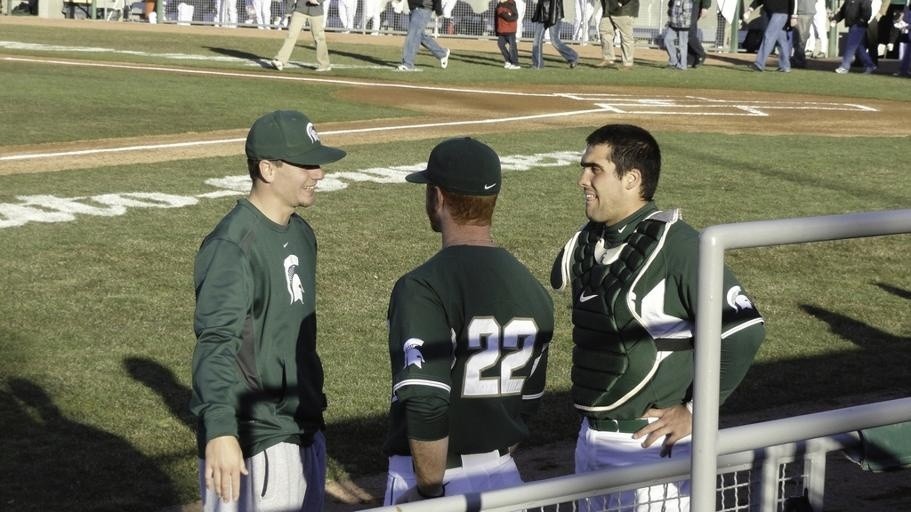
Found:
[584,413,647,434]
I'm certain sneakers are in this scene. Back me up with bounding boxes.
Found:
[440,49,450,68]
[395,64,409,71]
[271,60,283,71]
[315,67,331,71]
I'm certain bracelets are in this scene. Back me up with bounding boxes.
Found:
[415,484,448,499]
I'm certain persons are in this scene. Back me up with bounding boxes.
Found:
[386,132,556,512]
[323,1,409,36]
[396,0,450,70]
[188,108,347,512]
[144,0,296,32]
[548,122,766,511]
[432,0,497,37]
[664,0,711,69]
[272,0,330,71]
[495,0,640,68]
[739,0,909,74]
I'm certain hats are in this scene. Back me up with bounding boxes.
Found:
[405,136,500,195]
[246,111,347,165]
